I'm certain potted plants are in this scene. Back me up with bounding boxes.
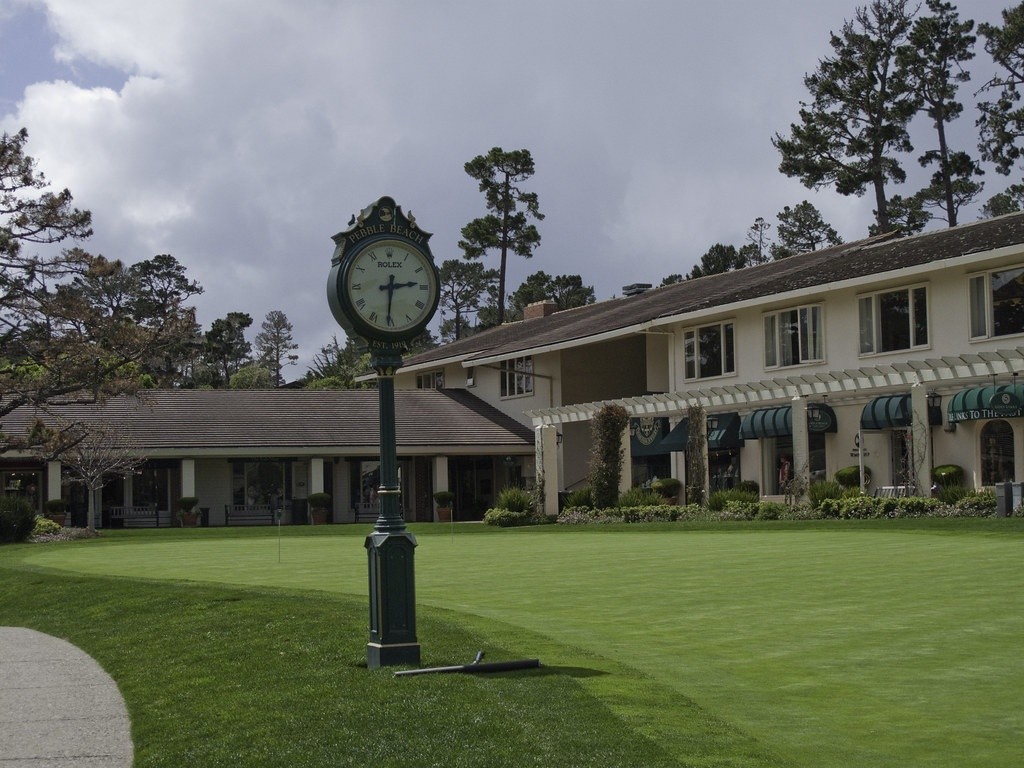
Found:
[650,477,681,506]
[308,492,332,524]
[434,491,454,521]
[46,499,67,528]
[180,497,199,528]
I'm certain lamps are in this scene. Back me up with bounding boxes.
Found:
[943,420,956,432]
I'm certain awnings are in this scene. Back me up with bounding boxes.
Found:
[860,392,942,429]
[948,381,1024,423]
[742,403,838,437]
[645,412,745,456]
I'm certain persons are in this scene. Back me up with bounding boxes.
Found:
[780,456,793,495]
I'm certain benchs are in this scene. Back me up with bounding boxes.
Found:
[354,502,380,523]
[873,486,915,498]
[108,506,159,527]
[224,504,275,526]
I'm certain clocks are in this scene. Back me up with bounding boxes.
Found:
[326,195,441,353]
